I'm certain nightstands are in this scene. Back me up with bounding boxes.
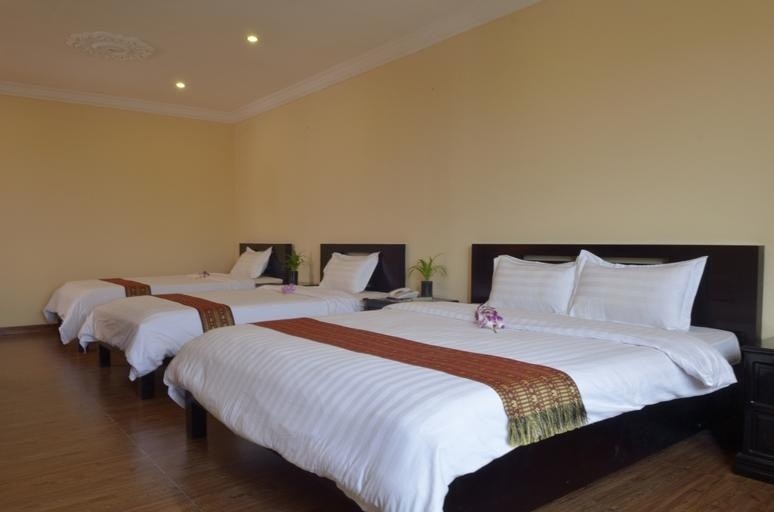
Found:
[733,336,774,486]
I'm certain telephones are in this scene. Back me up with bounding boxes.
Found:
[388,288,420,299]
